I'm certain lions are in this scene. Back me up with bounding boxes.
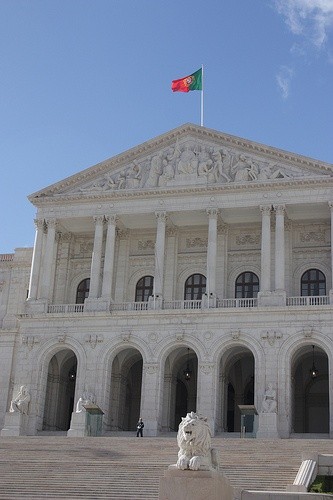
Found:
[174,411,218,471]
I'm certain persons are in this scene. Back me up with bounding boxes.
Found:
[10,386,31,415]
[261,382,277,413]
[75,397,89,414]
[136,417,144,437]
[104,144,288,190]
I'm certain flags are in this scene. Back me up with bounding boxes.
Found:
[171,67,202,93]
